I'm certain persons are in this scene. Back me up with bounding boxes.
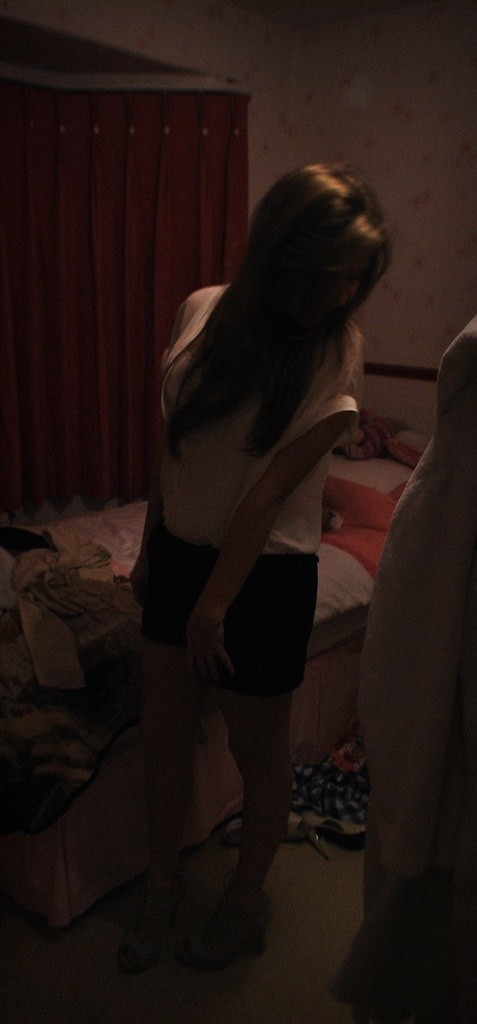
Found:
[114,163,391,972]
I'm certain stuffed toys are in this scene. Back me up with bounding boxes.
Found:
[345,410,431,468]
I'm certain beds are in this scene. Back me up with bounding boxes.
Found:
[0,449,416,942]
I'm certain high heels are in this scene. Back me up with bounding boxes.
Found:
[117,873,182,972]
[181,897,276,968]
[303,813,370,860]
[226,809,308,845]
